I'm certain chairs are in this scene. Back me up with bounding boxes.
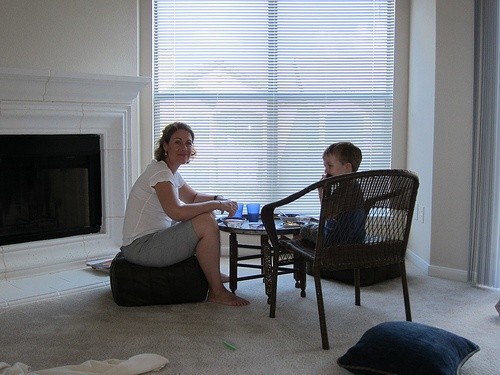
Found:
[261,169,419,349]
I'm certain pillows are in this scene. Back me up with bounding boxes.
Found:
[337,321,481,375]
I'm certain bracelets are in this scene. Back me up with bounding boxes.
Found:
[218,201,222,210]
[214,195,218,200]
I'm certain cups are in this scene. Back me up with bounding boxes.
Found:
[233,203,243,219]
[247,203,260,222]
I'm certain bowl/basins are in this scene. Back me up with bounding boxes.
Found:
[220,217,247,227]
[278,213,311,222]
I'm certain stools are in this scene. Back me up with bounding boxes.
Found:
[110,252,211,306]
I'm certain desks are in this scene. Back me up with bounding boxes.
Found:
[218,219,309,304]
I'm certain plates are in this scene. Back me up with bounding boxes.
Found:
[280,221,308,226]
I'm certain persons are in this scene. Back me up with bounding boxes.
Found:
[120,122,250,307]
[279,141,365,253]
